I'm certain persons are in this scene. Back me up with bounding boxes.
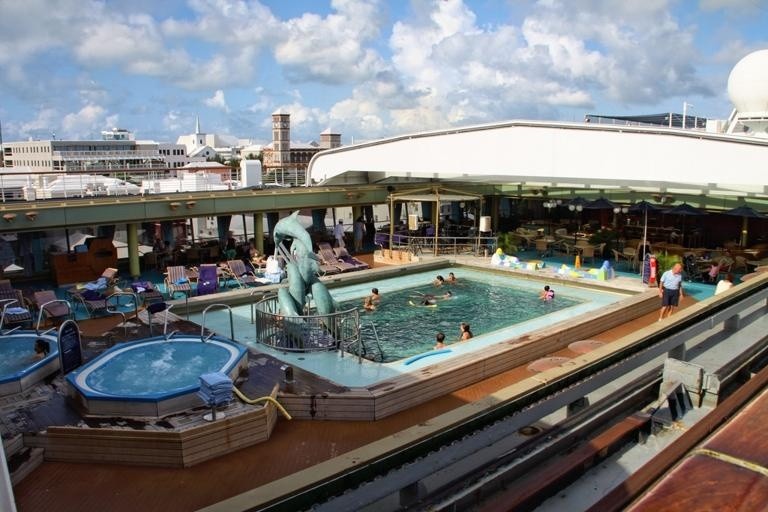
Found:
[539,286,550,301]
[725,249,734,262]
[715,272,735,295]
[460,322,473,341]
[30,339,51,363]
[435,275,444,286]
[658,263,684,321]
[447,272,456,283]
[443,291,452,300]
[635,234,651,276]
[371,288,380,303]
[363,296,375,311]
[433,333,446,350]
[153,216,376,264]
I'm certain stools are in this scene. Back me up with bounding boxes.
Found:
[202,402,228,422]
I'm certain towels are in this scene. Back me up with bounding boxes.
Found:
[196,371,233,404]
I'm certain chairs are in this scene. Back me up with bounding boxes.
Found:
[132,260,257,304]
[0,267,119,328]
[509,228,735,284]
[314,241,369,276]
